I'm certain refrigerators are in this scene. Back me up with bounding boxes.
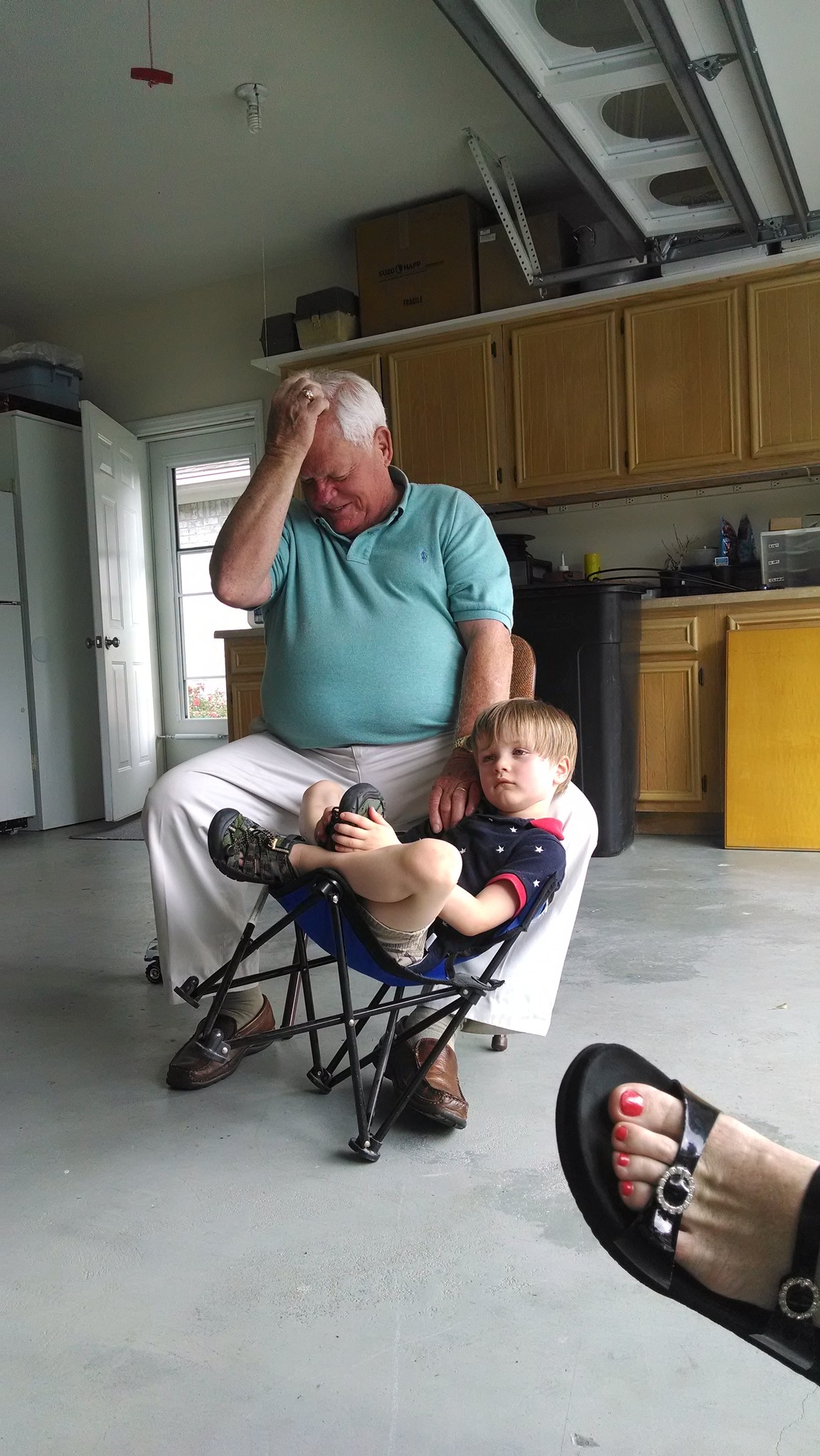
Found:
[0,490,36,836]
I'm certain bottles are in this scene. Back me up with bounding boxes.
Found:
[558,551,569,571]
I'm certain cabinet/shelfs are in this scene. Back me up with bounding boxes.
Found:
[634,597,820,835]
[760,526,820,588]
[213,631,269,743]
[281,259,819,506]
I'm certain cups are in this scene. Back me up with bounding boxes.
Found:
[695,549,718,566]
[584,554,601,582]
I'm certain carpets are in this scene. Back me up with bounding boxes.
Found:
[67,821,148,841]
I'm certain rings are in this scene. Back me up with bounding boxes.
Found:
[301,389,314,402]
[455,787,469,794]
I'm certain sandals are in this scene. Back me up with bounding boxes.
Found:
[208,807,307,887]
[315,781,387,851]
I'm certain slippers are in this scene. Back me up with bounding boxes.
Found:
[555,1041,820,1387]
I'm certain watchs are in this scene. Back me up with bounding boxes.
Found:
[453,734,472,752]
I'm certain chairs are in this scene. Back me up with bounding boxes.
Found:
[174,634,563,1164]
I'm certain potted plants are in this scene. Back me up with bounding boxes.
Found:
[657,524,699,598]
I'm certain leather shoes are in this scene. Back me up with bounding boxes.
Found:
[165,994,276,1090]
[372,1015,469,1130]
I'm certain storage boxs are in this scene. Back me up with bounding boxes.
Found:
[352,194,498,338]
[259,313,301,358]
[0,358,84,412]
[474,212,565,317]
[293,285,361,350]
[768,516,802,531]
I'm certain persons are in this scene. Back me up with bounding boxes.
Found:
[554,1042,820,1389]
[208,698,578,968]
[139,371,599,1130]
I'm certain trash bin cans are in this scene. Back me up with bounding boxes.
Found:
[507,583,642,860]
